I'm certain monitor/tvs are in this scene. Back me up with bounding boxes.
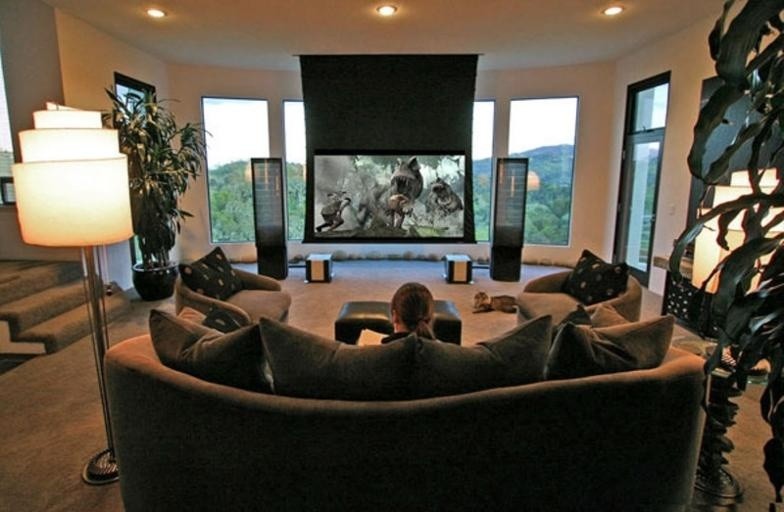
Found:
[311,148,468,241]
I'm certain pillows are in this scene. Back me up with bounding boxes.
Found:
[178,247,245,301]
[562,250,628,305]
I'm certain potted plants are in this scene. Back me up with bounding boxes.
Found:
[102,83,214,300]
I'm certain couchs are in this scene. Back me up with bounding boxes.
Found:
[103,332,714,509]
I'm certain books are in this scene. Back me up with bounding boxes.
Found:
[356,329,389,346]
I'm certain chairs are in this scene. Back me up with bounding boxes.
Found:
[516,272,642,322]
[175,267,292,323]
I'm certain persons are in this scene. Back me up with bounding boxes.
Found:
[315,197,351,232]
[386,194,409,230]
[378,283,436,344]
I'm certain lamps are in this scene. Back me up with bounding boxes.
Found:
[10,102,135,487]
